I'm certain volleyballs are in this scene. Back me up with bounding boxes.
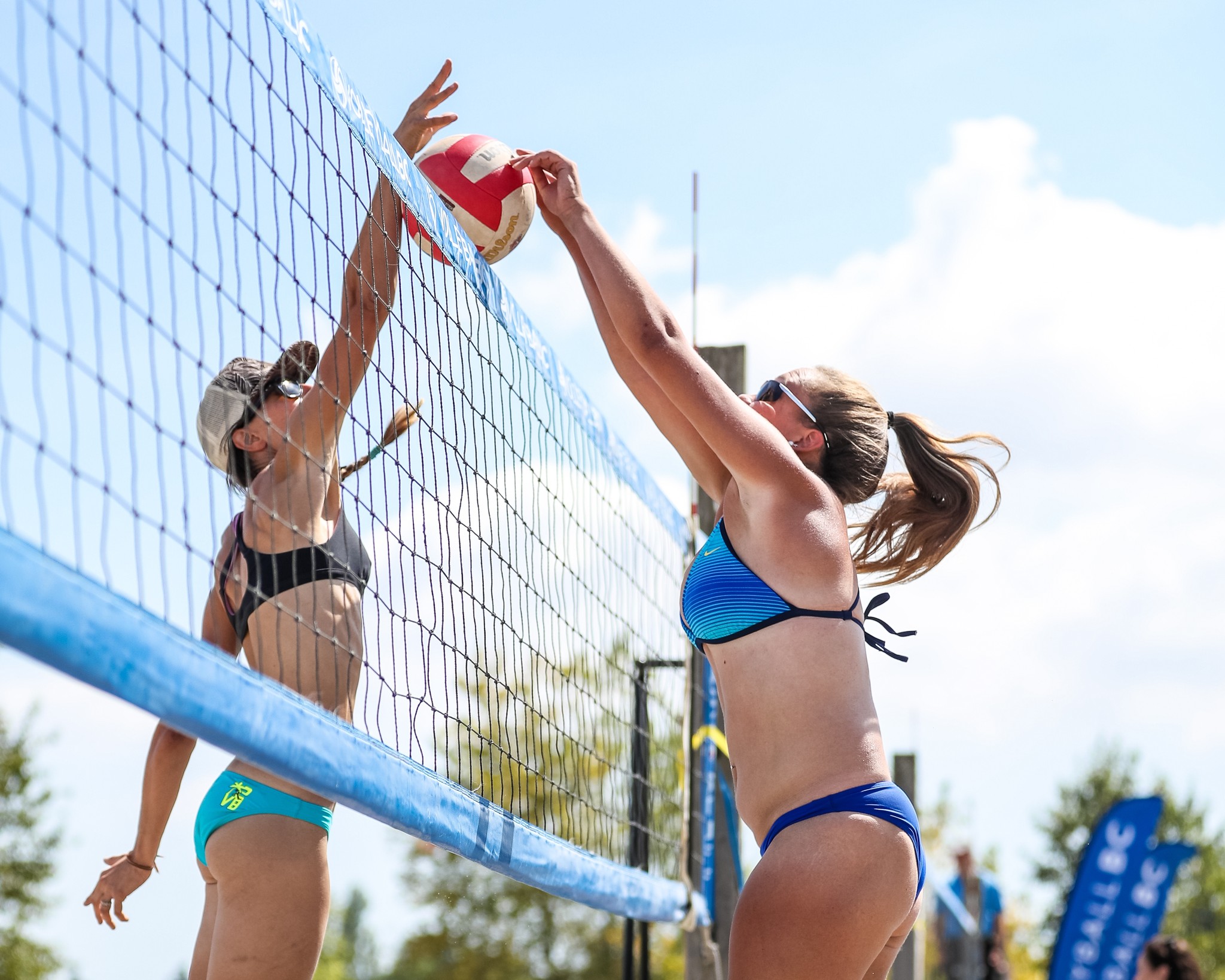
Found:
[400,131,538,267]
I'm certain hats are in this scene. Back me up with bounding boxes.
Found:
[196,340,319,473]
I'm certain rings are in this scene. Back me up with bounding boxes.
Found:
[99,900,112,906]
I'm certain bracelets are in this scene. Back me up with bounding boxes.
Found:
[124,852,165,872]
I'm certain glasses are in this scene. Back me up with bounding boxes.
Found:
[753,379,829,448]
[238,377,303,429]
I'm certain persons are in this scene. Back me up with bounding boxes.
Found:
[1131,934,1205,980]
[929,846,1008,980]
[509,149,1011,980]
[82,55,462,979]
